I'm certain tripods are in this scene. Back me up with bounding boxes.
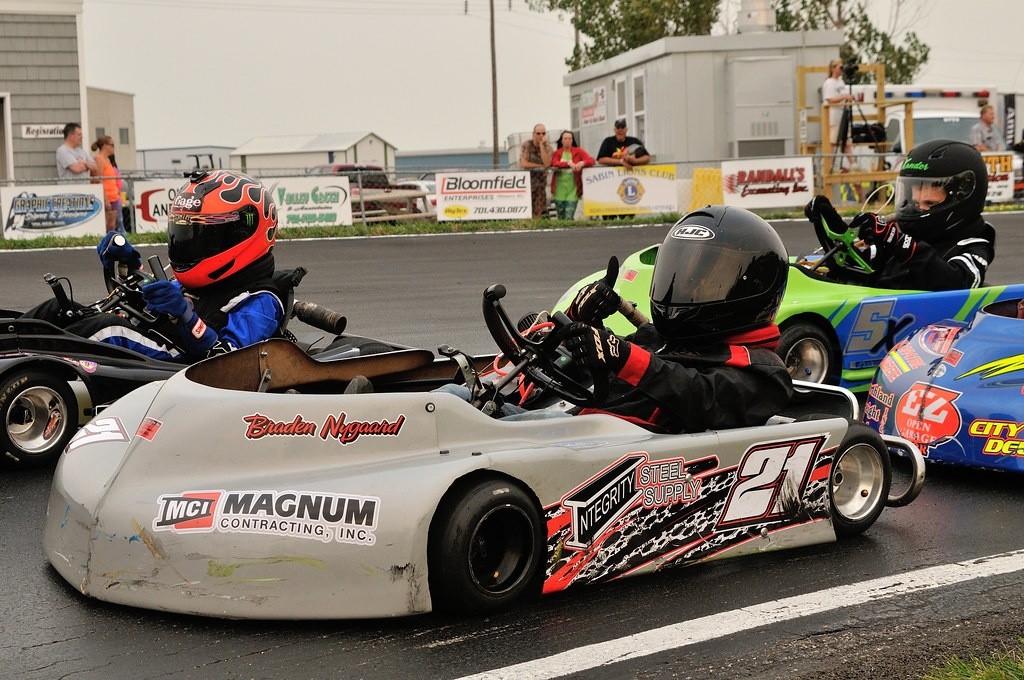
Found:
[830,77,882,176]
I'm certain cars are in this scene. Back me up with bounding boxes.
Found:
[392,180,440,214]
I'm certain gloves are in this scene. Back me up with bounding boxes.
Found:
[565,281,621,329]
[142,281,197,328]
[804,194,848,234]
[556,323,630,376]
[97,230,141,272]
[849,212,916,265]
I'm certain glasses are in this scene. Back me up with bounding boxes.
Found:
[535,132,545,135]
[616,126,625,129]
[105,142,114,147]
[840,67,842,69]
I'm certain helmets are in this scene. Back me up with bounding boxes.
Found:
[648,205,789,340]
[896,139,988,242]
[167,170,279,289]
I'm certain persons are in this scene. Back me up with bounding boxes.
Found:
[19,168,286,366]
[550,130,595,220]
[90,136,125,234]
[822,59,858,170]
[804,139,995,293]
[55,123,96,184]
[969,105,1001,206]
[428,205,792,435]
[520,124,553,217]
[596,118,650,220]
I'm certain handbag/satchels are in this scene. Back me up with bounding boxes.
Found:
[853,123,886,143]
[119,192,126,205]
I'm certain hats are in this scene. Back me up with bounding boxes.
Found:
[615,119,626,126]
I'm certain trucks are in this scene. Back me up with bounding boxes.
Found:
[827,81,1024,205]
[303,163,418,217]
